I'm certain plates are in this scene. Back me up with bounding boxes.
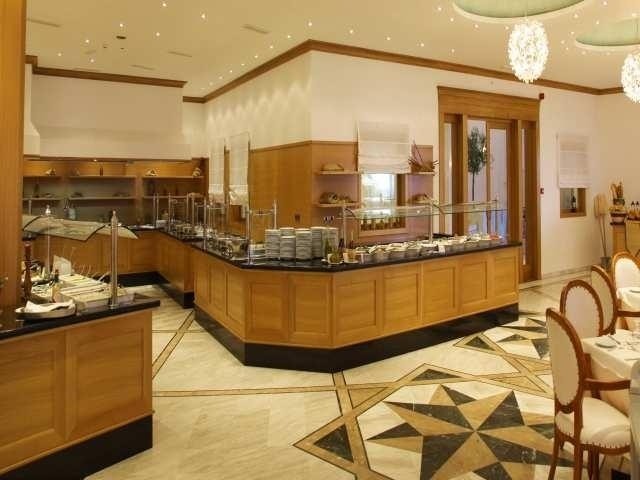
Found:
[330,259,359,266]
[631,288,640,293]
[263,227,338,261]
[597,330,639,358]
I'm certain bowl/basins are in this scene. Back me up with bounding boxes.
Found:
[14,303,77,319]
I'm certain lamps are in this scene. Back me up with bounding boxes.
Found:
[508,0,549,84]
[621,16,640,104]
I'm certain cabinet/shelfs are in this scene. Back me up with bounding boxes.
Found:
[316,169,364,207]
[626,220,640,259]
[22,153,210,226]
[406,170,438,203]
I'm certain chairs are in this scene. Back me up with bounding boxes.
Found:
[545,247,640,480]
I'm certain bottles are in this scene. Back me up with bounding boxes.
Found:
[45,204,51,214]
[572,196,577,208]
[631,201,640,220]
[337,192,352,203]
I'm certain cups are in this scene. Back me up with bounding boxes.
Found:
[343,248,358,261]
[416,194,424,201]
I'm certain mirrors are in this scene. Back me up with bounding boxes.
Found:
[358,171,409,237]
